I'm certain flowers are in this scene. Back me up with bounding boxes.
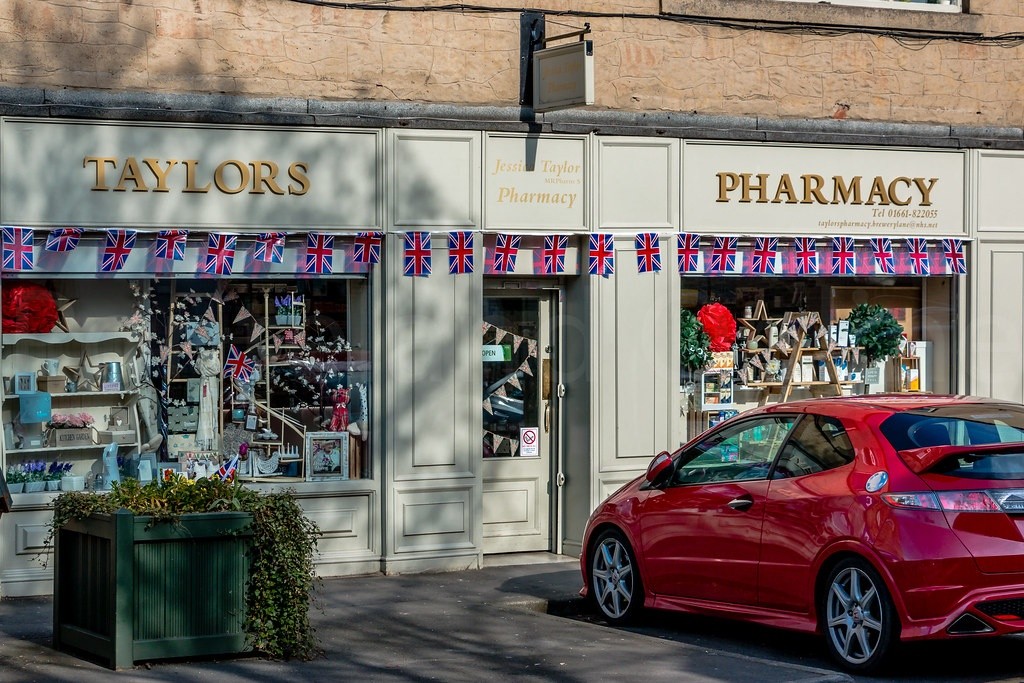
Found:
[44,411,94,429]
[162,468,232,495]
[5,458,75,484]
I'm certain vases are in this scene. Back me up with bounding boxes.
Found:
[46,429,93,448]
[7,482,24,493]
[46,480,61,491]
[23,481,46,492]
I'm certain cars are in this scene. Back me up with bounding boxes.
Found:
[578,391,1024,676]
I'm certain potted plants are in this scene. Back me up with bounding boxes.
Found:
[275,305,303,327]
[27,467,326,671]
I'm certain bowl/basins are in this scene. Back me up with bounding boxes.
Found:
[258,434,278,439]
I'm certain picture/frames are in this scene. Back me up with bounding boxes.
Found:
[132,453,158,482]
[306,431,350,481]
[178,452,221,480]
[15,372,34,394]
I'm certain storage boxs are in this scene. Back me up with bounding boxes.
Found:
[889,356,921,393]
[62,475,84,491]
[37,375,66,393]
[689,411,741,464]
[97,405,136,444]
[693,369,733,412]
[779,320,862,384]
[184,322,219,346]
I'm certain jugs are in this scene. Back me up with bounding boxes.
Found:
[103,442,121,489]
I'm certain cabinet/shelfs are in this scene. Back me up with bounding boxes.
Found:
[740,313,865,407]
[163,276,305,482]
[1,332,142,506]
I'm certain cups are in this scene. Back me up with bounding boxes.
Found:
[42,359,59,376]
[64,383,76,392]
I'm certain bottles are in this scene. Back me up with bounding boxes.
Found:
[234,408,244,421]
[745,306,752,318]
[774,283,783,314]
[699,279,736,314]
[719,411,738,422]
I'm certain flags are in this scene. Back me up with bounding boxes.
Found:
[869,238,894,273]
[45,228,85,251]
[588,234,614,274]
[795,237,817,274]
[353,232,384,263]
[711,237,738,271]
[224,346,254,380]
[2,227,34,270]
[404,232,432,275]
[752,238,779,273]
[544,235,567,273]
[206,233,237,275]
[101,230,137,271]
[255,232,287,263]
[448,232,474,274]
[636,233,662,272]
[831,237,854,274]
[306,233,334,274]
[677,234,700,271]
[943,239,967,273]
[155,230,188,261]
[493,233,521,272]
[906,238,929,274]
